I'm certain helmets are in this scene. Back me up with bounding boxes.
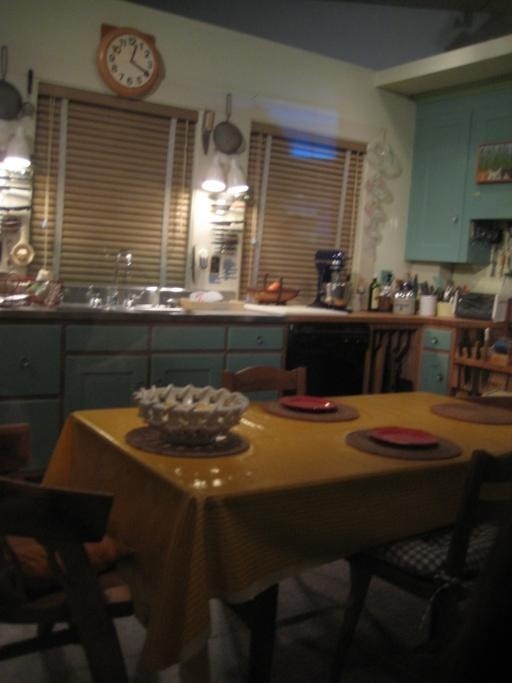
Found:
[5,294,31,300]
[368,424,441,448]
[279,393,338,413]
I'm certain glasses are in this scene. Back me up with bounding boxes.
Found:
[4,126,32,169]
[228,157,250,196]
[202,150,226,194]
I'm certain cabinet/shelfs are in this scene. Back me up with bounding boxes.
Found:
[367,275,381,311]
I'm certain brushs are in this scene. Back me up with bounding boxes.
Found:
[310,249,354,314]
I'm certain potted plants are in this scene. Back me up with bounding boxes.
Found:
[476,140,511,184]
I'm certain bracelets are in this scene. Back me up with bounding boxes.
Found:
[249,286,300,306]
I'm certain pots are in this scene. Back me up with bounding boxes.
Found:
[212,93,242,153]
[0,46,22,121]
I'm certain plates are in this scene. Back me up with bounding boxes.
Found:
[62,323,226,424]
[412,324,457,396]
[404,95,492,266]
[1,323,62,478]
[224,324,289,400]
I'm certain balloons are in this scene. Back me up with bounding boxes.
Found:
[97,22,162,98]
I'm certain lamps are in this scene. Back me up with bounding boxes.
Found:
[328,448,511,682]
[220,365,308,401]
[0,423,135,682]
